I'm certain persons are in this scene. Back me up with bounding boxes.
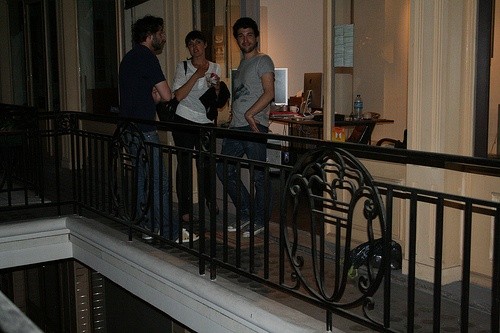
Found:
[217,18,274,237]
[170,29,221,226]
[119,15,199,243]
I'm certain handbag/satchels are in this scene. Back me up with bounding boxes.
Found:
[156,92,179,123]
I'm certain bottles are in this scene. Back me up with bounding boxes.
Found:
[209,73,219,85]
[353,95,362,117]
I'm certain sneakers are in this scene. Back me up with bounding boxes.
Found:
[241,221,264,237]
[227,217,250,232]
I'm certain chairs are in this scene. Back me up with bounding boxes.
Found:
[376,129,407,149]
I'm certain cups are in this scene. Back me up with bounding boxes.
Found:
[290,106,299,112]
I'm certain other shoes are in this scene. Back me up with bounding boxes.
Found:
[142,222,161,240]
[177,214,198,225]
[206,200,220,216]
[175,228,200,243]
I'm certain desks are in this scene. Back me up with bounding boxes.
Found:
[268,116,395,195]
